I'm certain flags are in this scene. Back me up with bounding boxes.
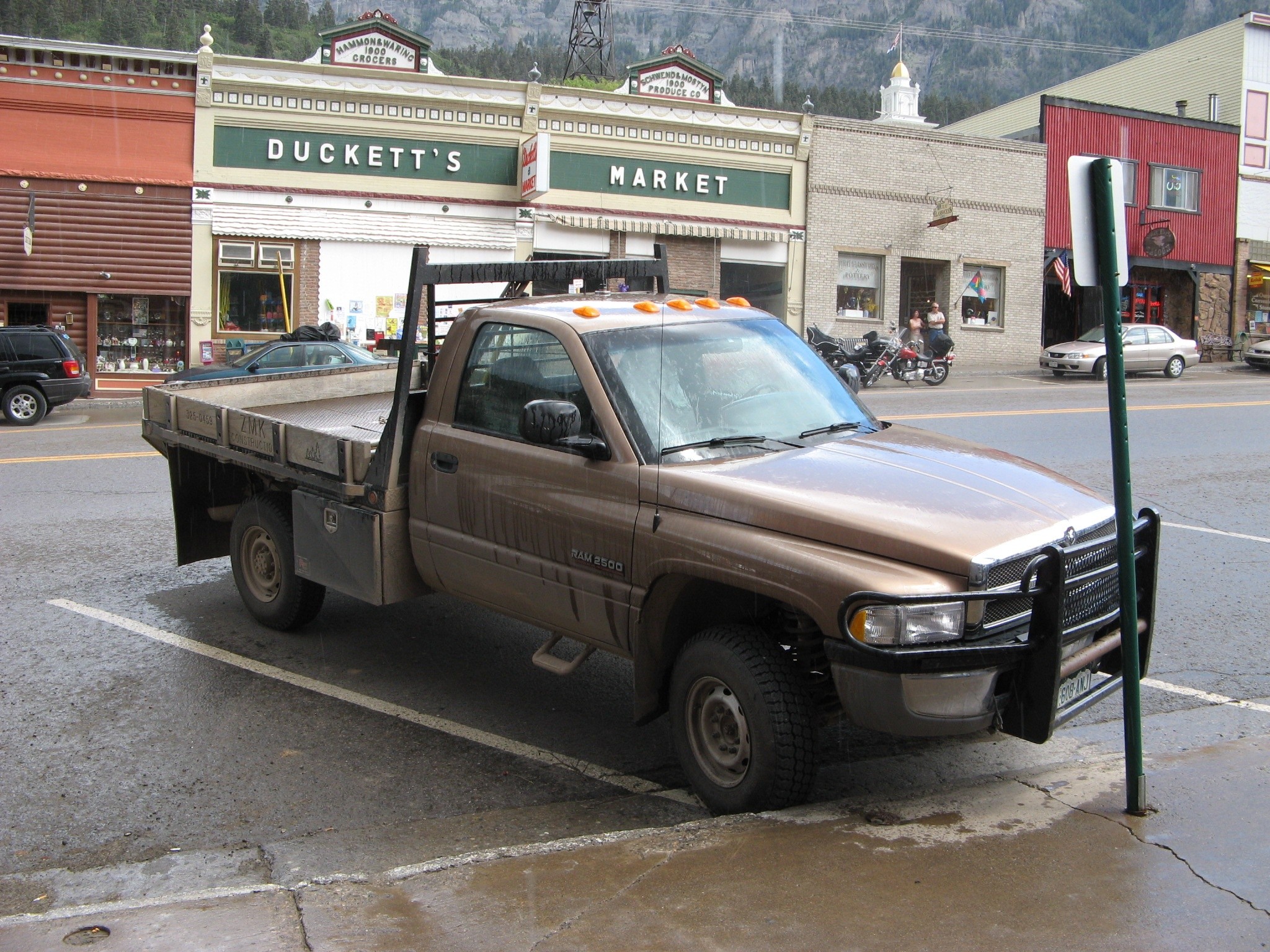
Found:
[969,270,986,304]
[887,32,900,54]
[1053,249,1071,297]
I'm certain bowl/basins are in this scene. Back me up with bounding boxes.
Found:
[129,362,139,368]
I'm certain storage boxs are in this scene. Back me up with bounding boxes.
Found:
[928,333,954,357]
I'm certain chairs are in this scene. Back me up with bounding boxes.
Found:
[475,355,561,436]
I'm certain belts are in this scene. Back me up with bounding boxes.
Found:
[931,328,943,331]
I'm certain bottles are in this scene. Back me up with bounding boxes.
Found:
[142,358,149,370]
[165,339,172,346]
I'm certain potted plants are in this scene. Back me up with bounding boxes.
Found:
[862,299,879,318]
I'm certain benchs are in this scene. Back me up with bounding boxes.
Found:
[1198,332,1243,363]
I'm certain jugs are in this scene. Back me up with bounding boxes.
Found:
[117,359,125,369]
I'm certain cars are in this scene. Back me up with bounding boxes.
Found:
[164,339,401,384]
[1039,323,1200,381]
[1244,339,1270,366]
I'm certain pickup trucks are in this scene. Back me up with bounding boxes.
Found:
[142,243,1160,820]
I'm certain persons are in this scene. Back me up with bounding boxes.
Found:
[927,303,946,343]
[909,309,926,355]
[838,286,873,317]
[976,300,986,318]
[964,298,975,319]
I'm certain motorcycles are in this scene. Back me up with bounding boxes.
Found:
[806,322,955,388]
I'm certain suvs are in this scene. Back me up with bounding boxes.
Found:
[0,324,92,426]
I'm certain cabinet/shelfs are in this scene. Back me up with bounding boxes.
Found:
[97,310,186,374]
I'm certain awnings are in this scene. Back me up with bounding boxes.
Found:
[1247,264,1270,280]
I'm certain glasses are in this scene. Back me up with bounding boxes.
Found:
[932,306,937,309]
